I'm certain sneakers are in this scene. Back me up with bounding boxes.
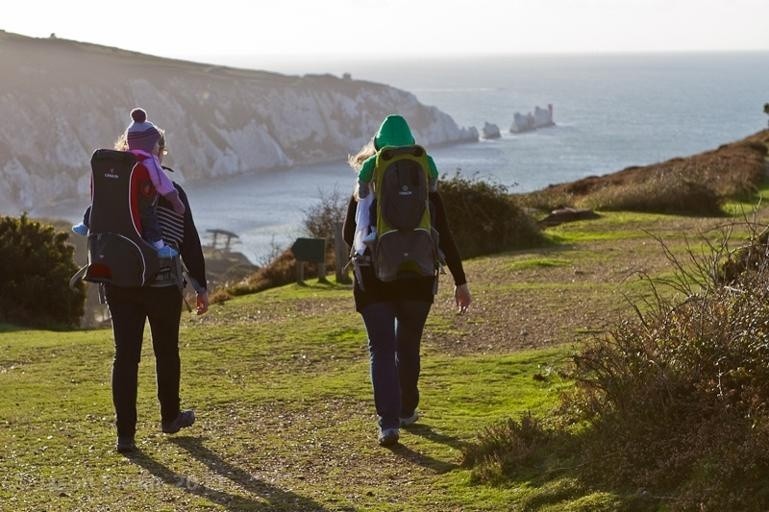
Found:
[73,223,87,236]
[158,245,177,258]
[117,438,134,449]
[399,412,418,427]
[363,231,376,245]
[378,428,398,446]
[162,411,194,433]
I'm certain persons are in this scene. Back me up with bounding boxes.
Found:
[341,142,471,444]
[87,121,207,452]
[72,108,186,262]
[357,114,438,268]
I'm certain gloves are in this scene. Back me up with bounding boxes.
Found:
[165,190,187,214]
[359,181,368,198]
[430,179,437,193]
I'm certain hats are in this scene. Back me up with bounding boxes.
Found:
[127,109,160,154]
